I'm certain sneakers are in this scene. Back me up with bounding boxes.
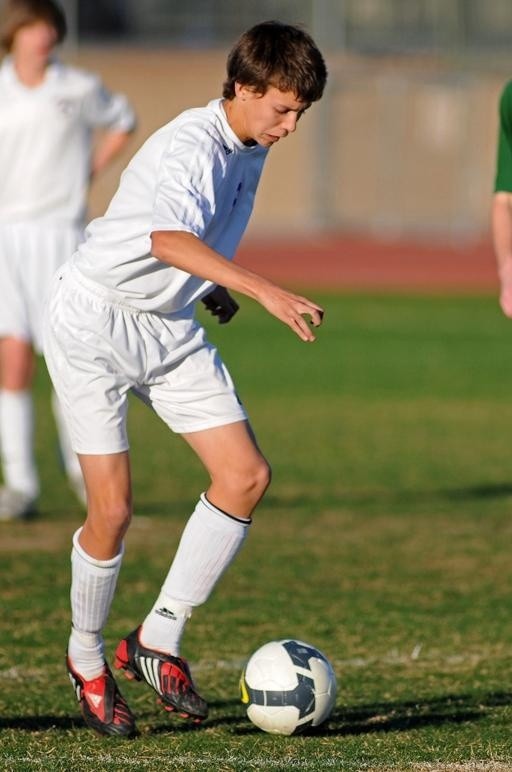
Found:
[113,624,209,724]
[0,488,39,522]
[66,648,137,740]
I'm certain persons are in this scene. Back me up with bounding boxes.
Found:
[491,79,512,318]
[0,0,136,526]
[41,21,328,742]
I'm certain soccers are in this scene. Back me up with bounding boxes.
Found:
[239,639,337,737]
